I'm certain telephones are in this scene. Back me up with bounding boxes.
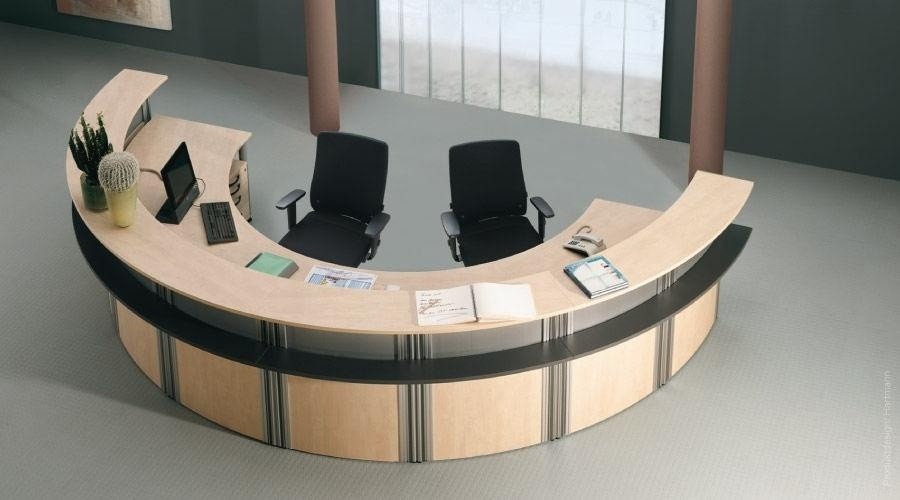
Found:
[562,233,606,256]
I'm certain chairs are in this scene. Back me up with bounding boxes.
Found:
[441,139,555,268]
[275,132,390,268]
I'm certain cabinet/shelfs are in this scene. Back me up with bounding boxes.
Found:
[229,160,251,221]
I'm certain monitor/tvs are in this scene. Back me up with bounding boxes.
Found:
[155,141,200,224]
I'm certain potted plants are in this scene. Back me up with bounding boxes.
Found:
[68,111,114,213]
[98,152,139,228]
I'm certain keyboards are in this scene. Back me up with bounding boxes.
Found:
[200,202,238,245]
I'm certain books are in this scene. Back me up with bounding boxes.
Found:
[563,254,629,299]
[415,282,540,327]
[248,253,298,278]
